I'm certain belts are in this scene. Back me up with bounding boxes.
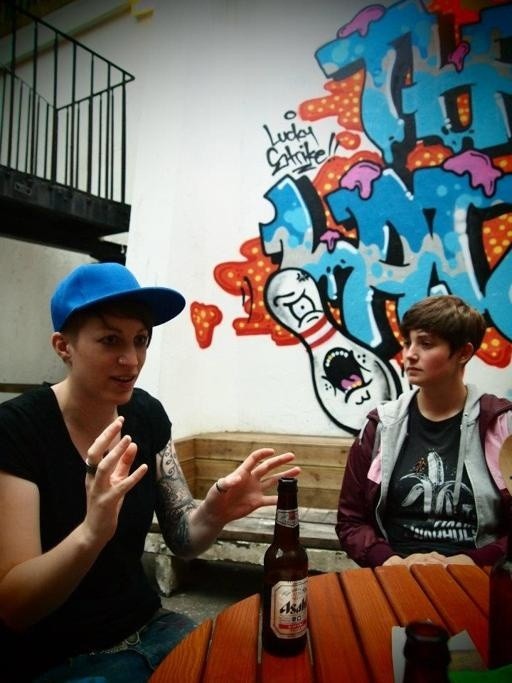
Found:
[95,631,140,655]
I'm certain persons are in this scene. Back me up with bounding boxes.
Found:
[336,295,511,569]
[1,262,304,683]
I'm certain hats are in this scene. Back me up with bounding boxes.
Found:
[51,263,185,332]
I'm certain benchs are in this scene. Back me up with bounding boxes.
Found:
[143,432,370,597]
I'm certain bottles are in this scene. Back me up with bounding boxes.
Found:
[486,509,512,672]
[263,476,312,656]
[402,617,454,682]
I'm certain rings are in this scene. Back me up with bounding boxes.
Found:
[215,483,227,497]
[84,458,98,475]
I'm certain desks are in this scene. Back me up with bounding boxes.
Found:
[150,564,488,683]
